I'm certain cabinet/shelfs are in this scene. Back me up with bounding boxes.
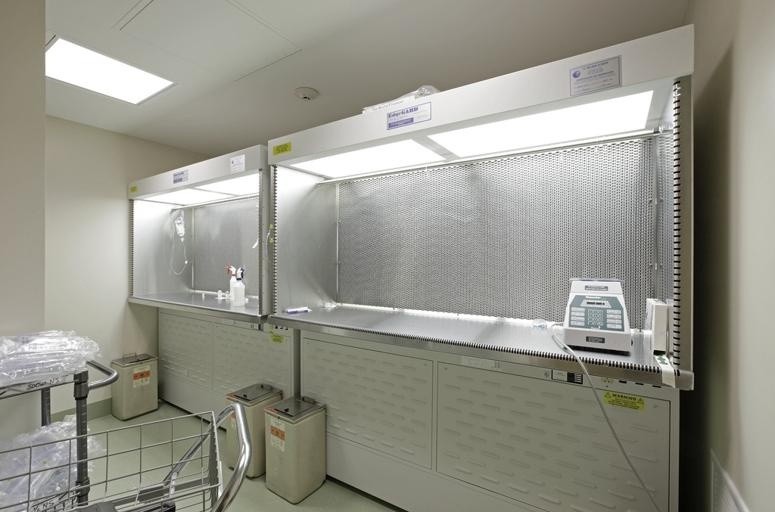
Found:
[266,25,692,512]
[126,143,301,431]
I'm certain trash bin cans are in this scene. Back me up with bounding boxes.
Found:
[263,396,327,504]
[111,353,158,421]
[225,383,283,480]
